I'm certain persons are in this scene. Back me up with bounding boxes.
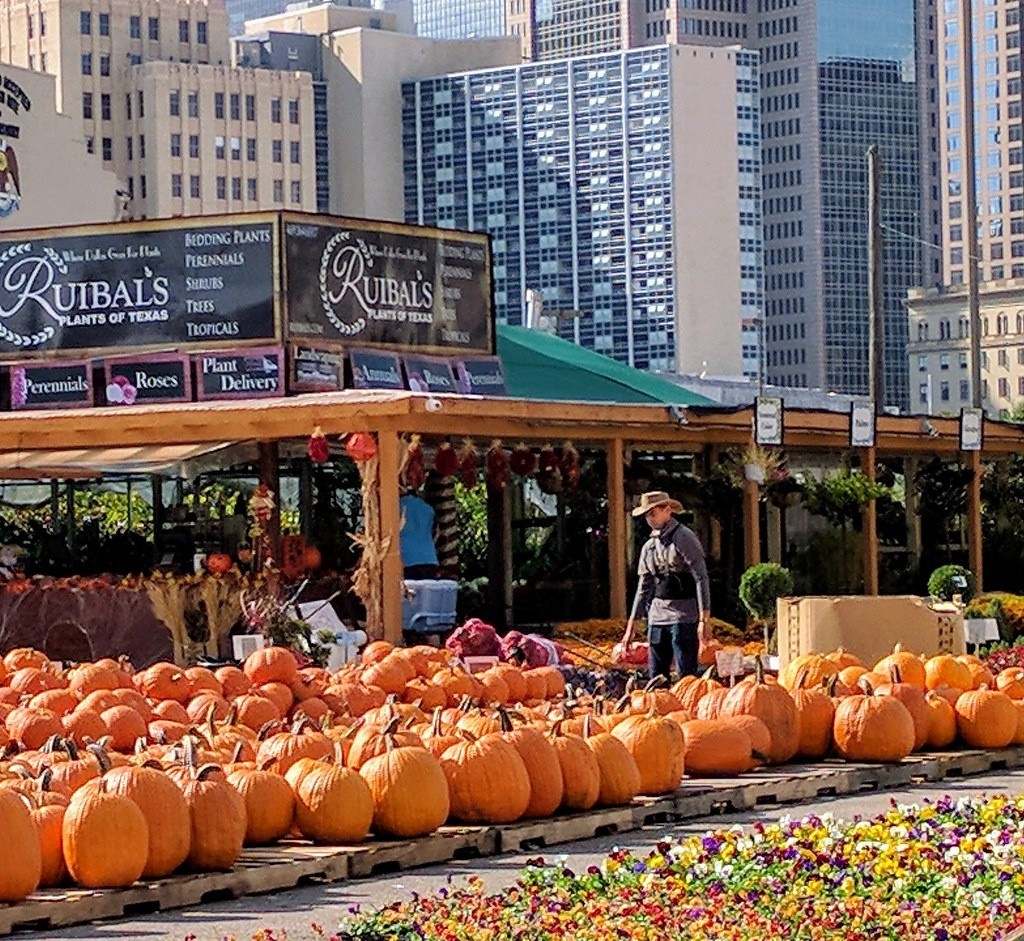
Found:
[400,491,440,646]
[622,492,710,684]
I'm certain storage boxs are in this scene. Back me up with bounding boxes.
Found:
[778,597,968,683]
[399,579,460,630]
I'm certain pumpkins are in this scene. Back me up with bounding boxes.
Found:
[206,553,232,575]
[0,640,1024,902]
[304,546,321,570]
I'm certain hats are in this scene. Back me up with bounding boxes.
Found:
[632,491,683,516]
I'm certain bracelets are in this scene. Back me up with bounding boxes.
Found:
[699,618,708,622]
